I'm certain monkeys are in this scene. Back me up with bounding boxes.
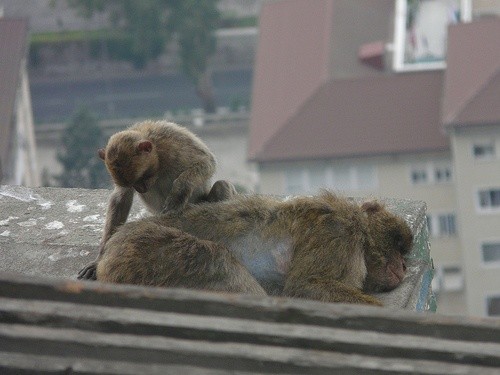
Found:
[78,121,413,307]
